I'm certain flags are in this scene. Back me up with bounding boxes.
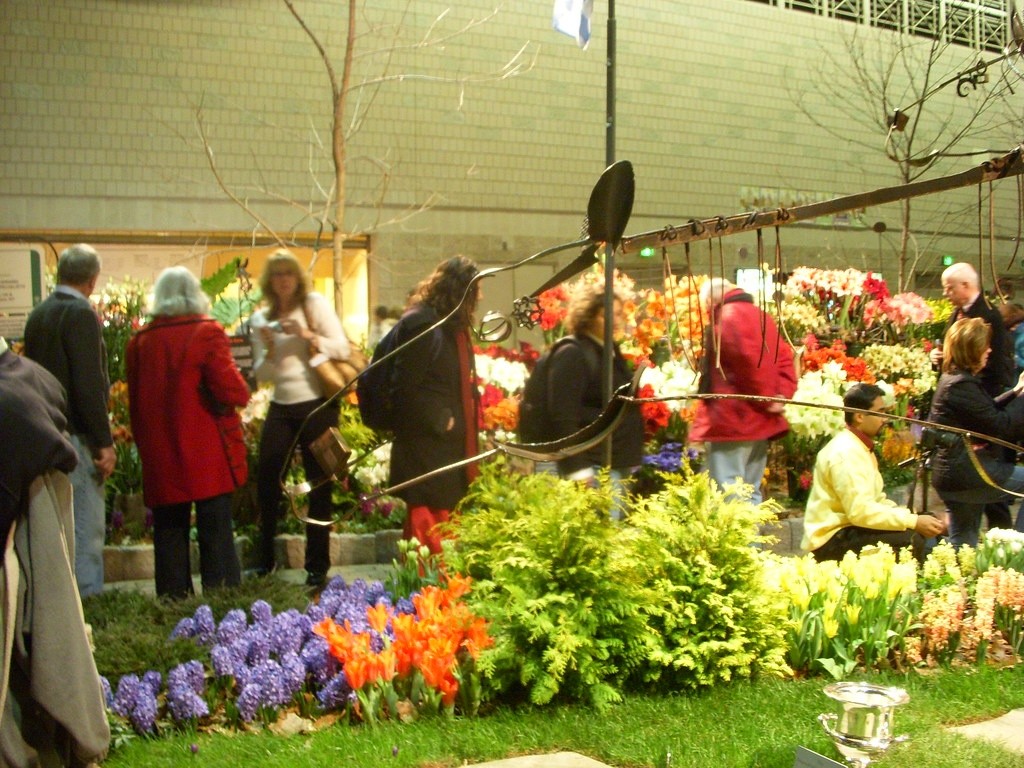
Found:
[551,0,593,51]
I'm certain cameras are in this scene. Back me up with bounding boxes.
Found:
[267,322,282,334]
[918,427,961,453]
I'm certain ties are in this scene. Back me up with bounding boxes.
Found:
[957,307,963,320]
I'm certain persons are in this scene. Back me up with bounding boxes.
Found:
[125,265,251,600]
[387,255,482,585]
[546,284,645,522]
[252,249,350,586]
[924,262,1024,564]
[23,242,117,600]
[0,336,111,768]
[800,384,944,570]
[689,278,798,553]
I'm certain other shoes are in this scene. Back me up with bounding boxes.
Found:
[243,567,268,578]
[304,571,327,585]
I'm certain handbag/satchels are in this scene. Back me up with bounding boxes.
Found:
[299,297,370,399]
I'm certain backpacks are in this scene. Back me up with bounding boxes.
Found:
[518,334,623,452]
[357,307,444,432]
